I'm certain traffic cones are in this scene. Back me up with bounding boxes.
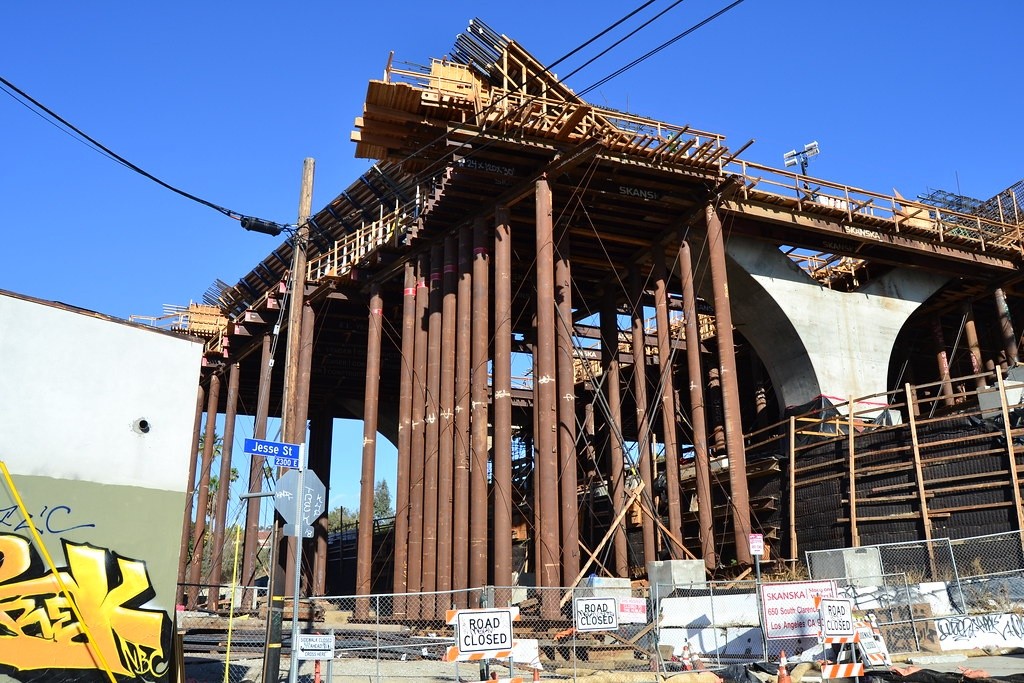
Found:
[778,650,791,683]
[682,646,693,671]
[688,642,706,671]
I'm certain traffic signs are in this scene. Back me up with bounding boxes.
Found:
[243,438,300,460]
[274,457,299,469]
